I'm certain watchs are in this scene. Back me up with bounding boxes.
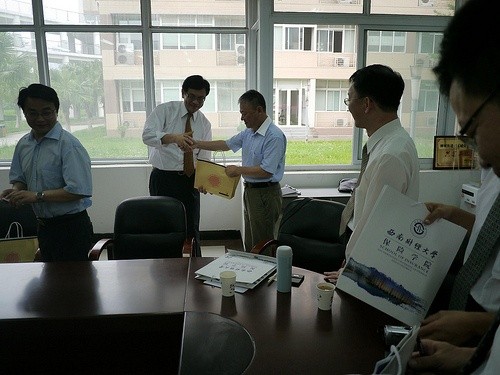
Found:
[36,191,44,203]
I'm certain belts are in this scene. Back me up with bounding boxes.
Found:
[37,209,86,226]
[156,168,185,176]
[243,181,279,188]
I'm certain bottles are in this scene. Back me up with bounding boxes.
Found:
[276,245,293,293]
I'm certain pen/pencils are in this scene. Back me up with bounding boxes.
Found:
[267,273,277,285]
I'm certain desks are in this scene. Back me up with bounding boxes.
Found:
[274,188,352,240]
[0,258,385,375]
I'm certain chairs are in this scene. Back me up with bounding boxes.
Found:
[0,180,354,273]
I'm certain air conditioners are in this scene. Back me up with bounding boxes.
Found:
[336,118,348,126]
[418,0,433,7]
[115,43,135,65]
[335,57,349,67]
[123,120,135,128]
[427,117,435,127]
[236,44,244,65]
[414,56,432,67]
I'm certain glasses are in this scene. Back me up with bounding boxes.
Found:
[456,89,500,150]
[22,107,56,119]
[184,92,206,103]
[344,96,372,106]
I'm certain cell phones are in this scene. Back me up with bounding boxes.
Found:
[291,274,304,286]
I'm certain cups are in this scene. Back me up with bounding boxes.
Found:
[316,283,336,310]
[220,270,237,297]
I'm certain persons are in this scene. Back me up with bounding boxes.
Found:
[142,75,211,257]
[418,167,499,348]
[0,83,93,263]
[178,89,286,258]
[324,64,420,280]
[409,1,500,375]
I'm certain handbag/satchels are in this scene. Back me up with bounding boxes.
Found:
[0,222,39,263]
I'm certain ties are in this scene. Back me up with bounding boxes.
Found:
[448,192,500,312]
[339,142,368,236]
[183,113,194,178]
